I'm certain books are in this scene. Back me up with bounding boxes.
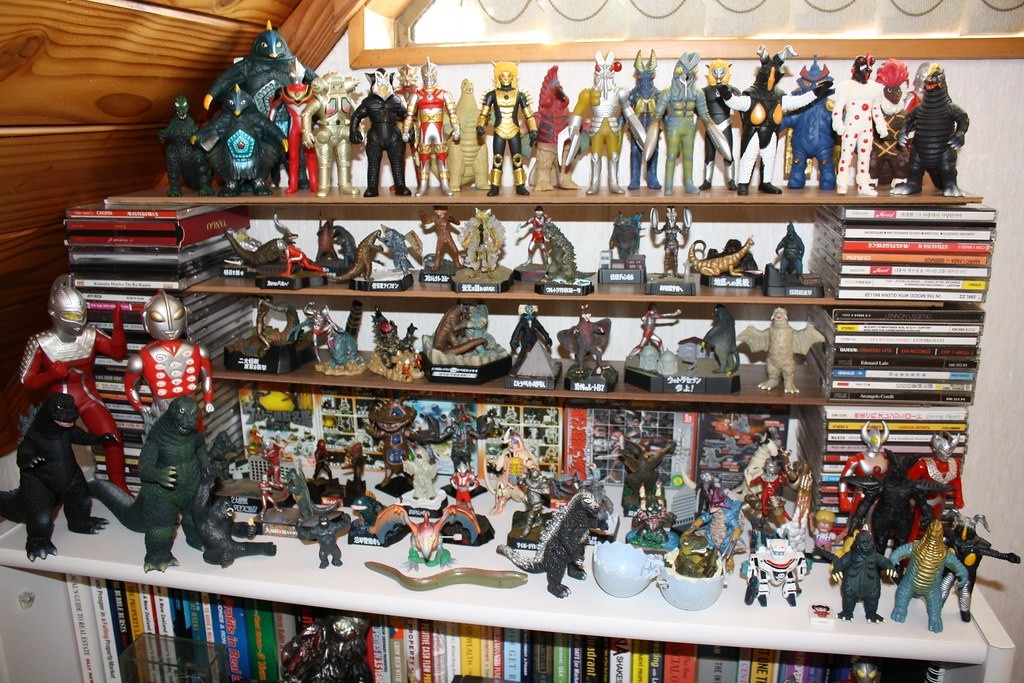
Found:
[67,574,947,683]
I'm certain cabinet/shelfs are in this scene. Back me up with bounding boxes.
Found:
[0,182,1015,683]
